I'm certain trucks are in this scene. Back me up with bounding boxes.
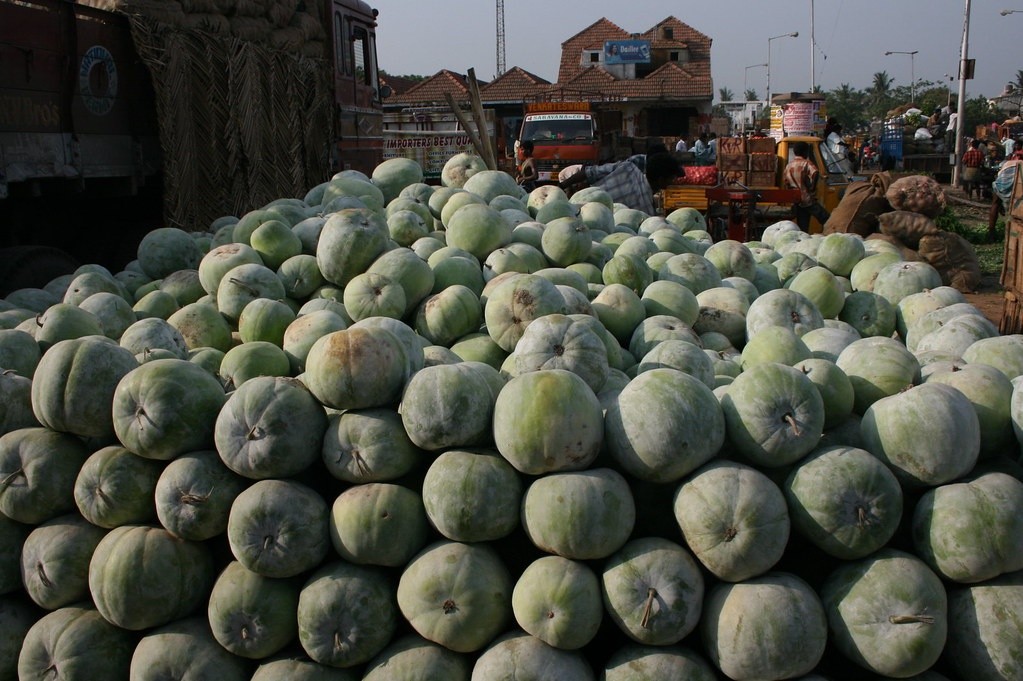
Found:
[879,116,953,177]
[1,0,392,199]
[664,133,852,240]
[512,112,602,192]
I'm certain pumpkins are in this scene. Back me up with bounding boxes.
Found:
[0,155,1023,681]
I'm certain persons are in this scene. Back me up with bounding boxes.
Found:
[826,117,881,173]
[984,160,1023,235]
[515,141,539,193]
[784,141,830,233]
[927,101,1023,199]
[676,132,717,155]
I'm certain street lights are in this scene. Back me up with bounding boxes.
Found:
[943,74,953,106]
[768,32,800,106]
[744,63,768,101]
[884,50,918,103]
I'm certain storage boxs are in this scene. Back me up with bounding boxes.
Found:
[0,0,150,134]
[713,137,778,191]
[996,159,1023,296]
